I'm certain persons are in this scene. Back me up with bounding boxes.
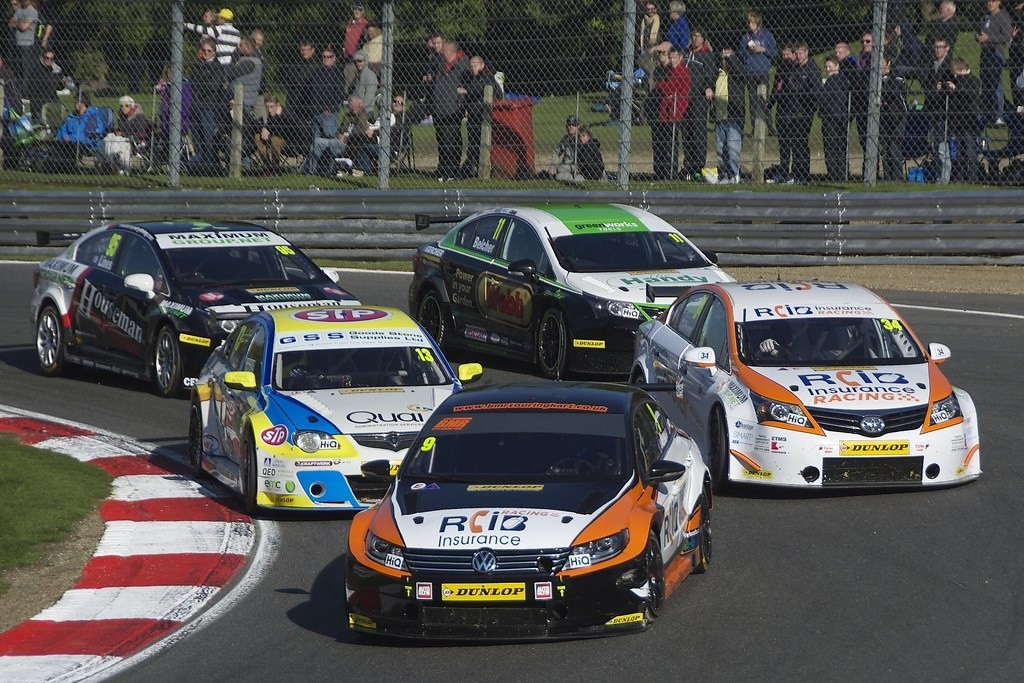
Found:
[817,56,852,182]
[115,95,148,151]
[255,4,411,179]
[423,35,474,179]
[53,91,106,161]
[734,9,776,136]
[639,1,691,65]
[552,113,609,181]
[456,55,502,179]
[835,0,1024,185]
[757,320,792,364]
[702,44,749,186]
[767,43,823,185]
[652,31,721,182]
[155,7,288,171]
[0,0,65,128]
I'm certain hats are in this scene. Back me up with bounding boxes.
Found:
[216,8,234,21]
[350,0,363,9]
[566,115,580,124]
[691,29,706,42]
[353,54,363,61]
[118,96,131,105]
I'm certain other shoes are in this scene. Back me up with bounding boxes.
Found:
[719,175,739,184]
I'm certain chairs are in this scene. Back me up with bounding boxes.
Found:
[94,105,114,123]
[390,126,416,177]
[41,102,65,129]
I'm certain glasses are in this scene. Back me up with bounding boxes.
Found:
[43,56,53,61]
[393,100,403,105]
[322,55,333,58]
[861,39,871,44]
[202,49,213,52]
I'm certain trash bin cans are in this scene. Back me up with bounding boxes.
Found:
[490,94,539,177]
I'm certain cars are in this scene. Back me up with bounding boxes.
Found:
[409,201,738,377]
[631,283,982,491]
[183,304,481,516]
[27,216,361,398]
[346,381,716,637]
